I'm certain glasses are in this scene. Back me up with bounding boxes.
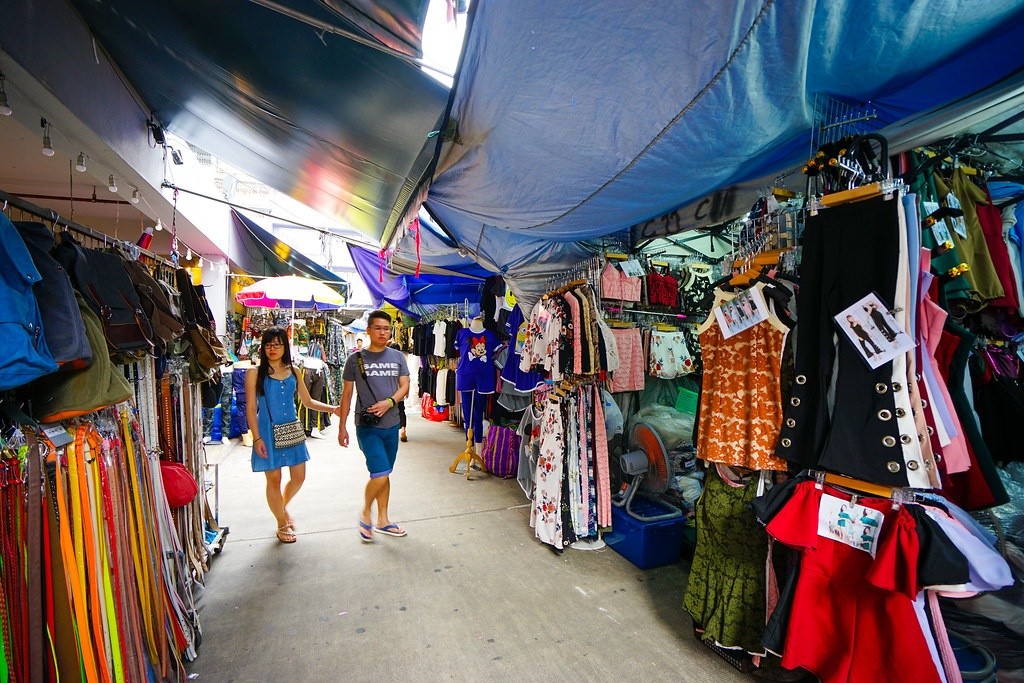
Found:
[263,342,284,350]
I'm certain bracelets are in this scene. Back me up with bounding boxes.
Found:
[386,397,394,407]
[389,397,395,406]
[254,437,261,443]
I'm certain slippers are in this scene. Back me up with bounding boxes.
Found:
[358,520,375,543]
[283,512,296,532]
[276,524,296,543]
[374,523,407,537]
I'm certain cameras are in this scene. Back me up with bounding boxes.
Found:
[361,406,380,425]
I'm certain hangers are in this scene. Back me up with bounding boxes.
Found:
[415,306,463,371]
[249,306,344,347]
[535,114,914,509]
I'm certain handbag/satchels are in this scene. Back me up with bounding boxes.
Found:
[158,436,199,508]
[482,422,522,480]
[272,420,307,449]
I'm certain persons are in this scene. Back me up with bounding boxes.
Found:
[245,326,350,542]
[353,338,362,352]
[339,310,407,541]
[724,296,759,333]
[847,315,885,358]
[390,342,410,442]
[203,357,325,445]
[863,302,898,346]
[455,319,500,467]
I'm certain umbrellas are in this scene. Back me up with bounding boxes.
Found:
[236,275,345,363]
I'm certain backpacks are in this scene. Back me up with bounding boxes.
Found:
[0,205,234,424]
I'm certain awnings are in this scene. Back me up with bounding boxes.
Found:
[73,1,453,297]
